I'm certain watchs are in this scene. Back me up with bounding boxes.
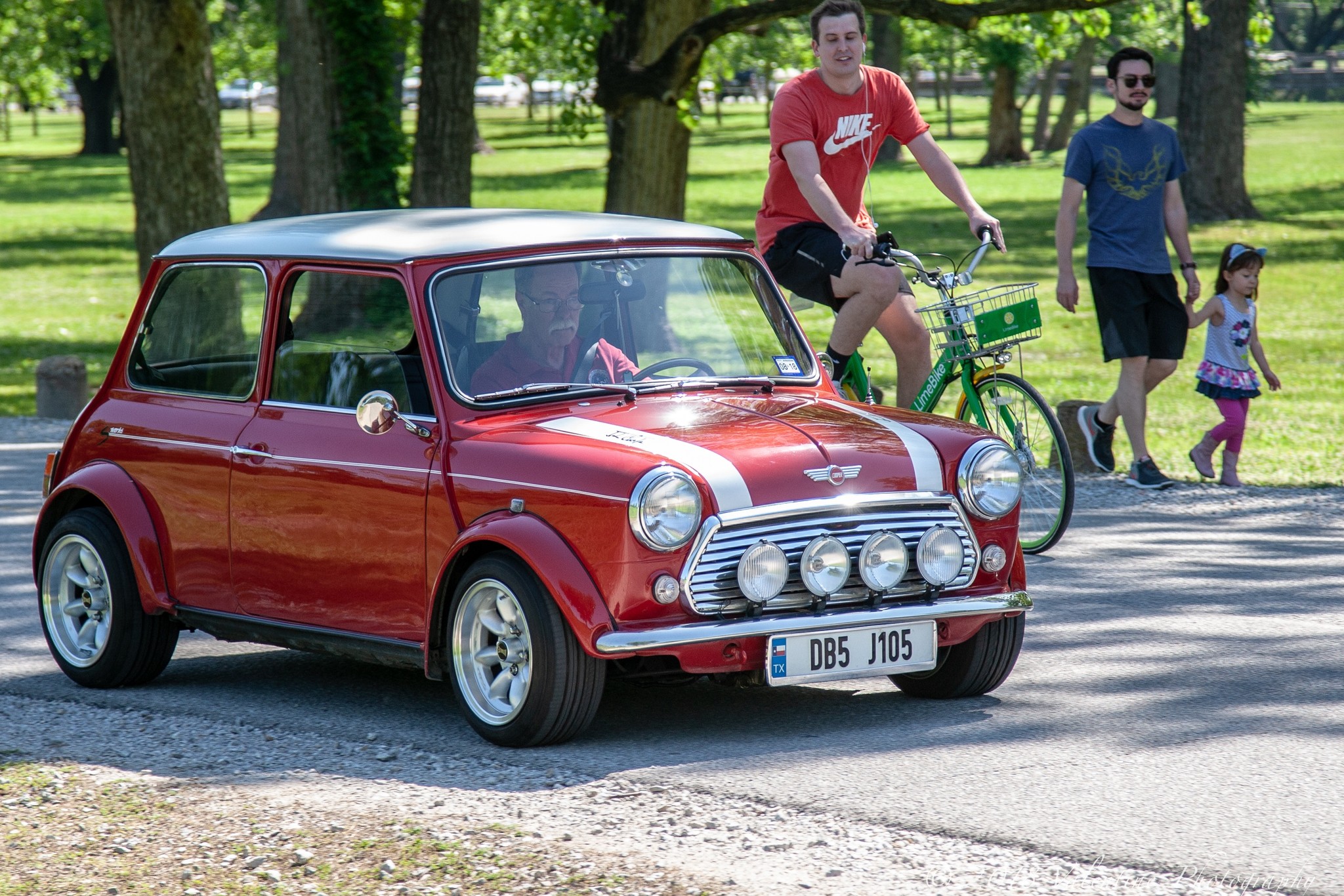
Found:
[1179,261,1198,271]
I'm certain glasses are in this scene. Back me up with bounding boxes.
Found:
[1114,73,1156,88]
[519,289,585,313]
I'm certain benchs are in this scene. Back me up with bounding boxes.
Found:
[153,359,249,395]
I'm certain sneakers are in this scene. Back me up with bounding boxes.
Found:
[1123,455,1176,488]
[1078,403,1115,473]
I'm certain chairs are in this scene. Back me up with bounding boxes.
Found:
[456,339,503,392]
[328,351,431,415]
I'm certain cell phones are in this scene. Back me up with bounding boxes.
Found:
[876,231,900,249]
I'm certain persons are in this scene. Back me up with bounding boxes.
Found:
[756,0,1005,413]
[468,262,658,395]
[1051,48,1201,489]
[1182,243,1283,488]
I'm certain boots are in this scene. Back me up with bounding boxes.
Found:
[1220,450,1246,488]
[1189,430,1218,478]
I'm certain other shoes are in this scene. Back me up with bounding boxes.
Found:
[834,371,851,401]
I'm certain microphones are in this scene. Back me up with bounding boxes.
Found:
[862,42,866,52]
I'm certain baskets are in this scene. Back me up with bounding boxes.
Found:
[915,282,1043,360]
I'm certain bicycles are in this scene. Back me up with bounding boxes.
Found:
[839,222,1079,555]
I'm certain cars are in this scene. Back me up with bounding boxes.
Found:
[216,77,269,110]
[401,64,777,110]
[28,206,1037,750]
